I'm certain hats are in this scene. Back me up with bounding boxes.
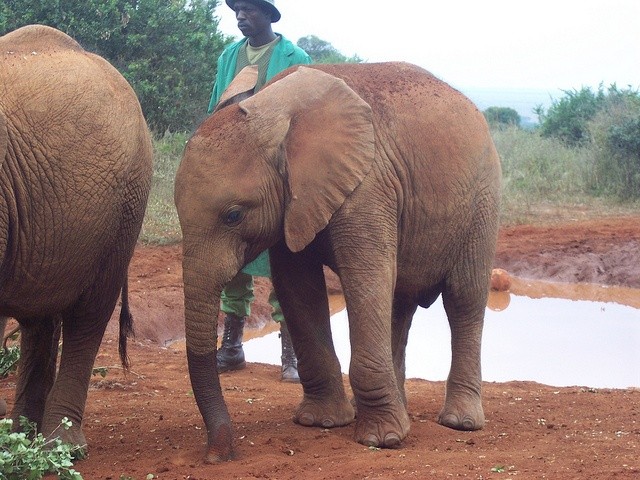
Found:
[226,0,280,22]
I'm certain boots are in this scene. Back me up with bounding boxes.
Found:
[217,312,245,373]
[279,322,301,383]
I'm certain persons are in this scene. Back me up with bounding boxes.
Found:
[202,0,313,384]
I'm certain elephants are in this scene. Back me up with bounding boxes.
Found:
[0,22,157,459]
[173,56,505,466]
